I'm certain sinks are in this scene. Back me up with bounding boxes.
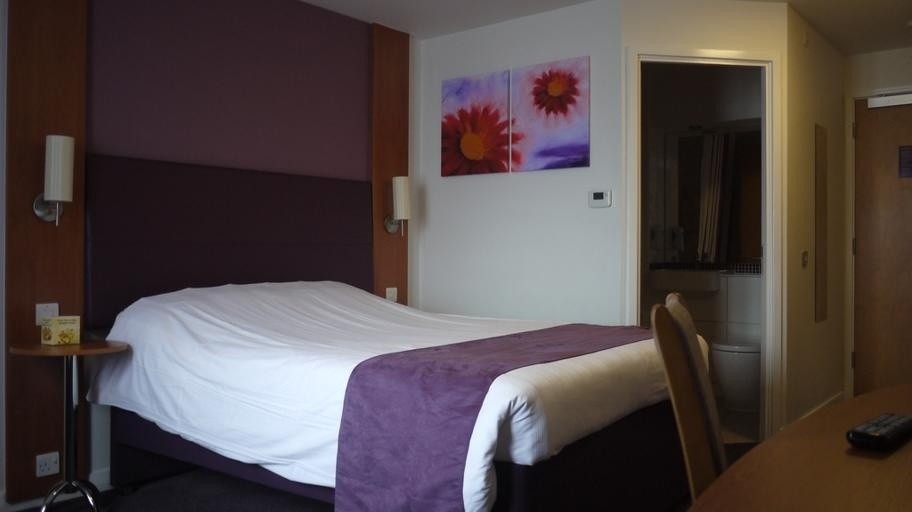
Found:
[650,269,719,291]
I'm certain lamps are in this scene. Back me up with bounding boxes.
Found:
[384,176,412,237]
[32,135,76,228]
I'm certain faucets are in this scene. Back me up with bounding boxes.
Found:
[678,224,686,268]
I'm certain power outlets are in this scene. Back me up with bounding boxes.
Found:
[36,451,60,479]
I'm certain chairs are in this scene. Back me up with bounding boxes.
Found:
[650,293,725,502]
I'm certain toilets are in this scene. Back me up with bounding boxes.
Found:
[709,342,760,414]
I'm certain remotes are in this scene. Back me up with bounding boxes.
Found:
[846,413,912,447]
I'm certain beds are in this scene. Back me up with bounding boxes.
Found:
[84,148,711,511]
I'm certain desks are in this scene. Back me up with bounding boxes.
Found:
[11,336,133,511]
[688,383,911,511]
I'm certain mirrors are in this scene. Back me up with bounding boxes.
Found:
[646,130,761,265]
